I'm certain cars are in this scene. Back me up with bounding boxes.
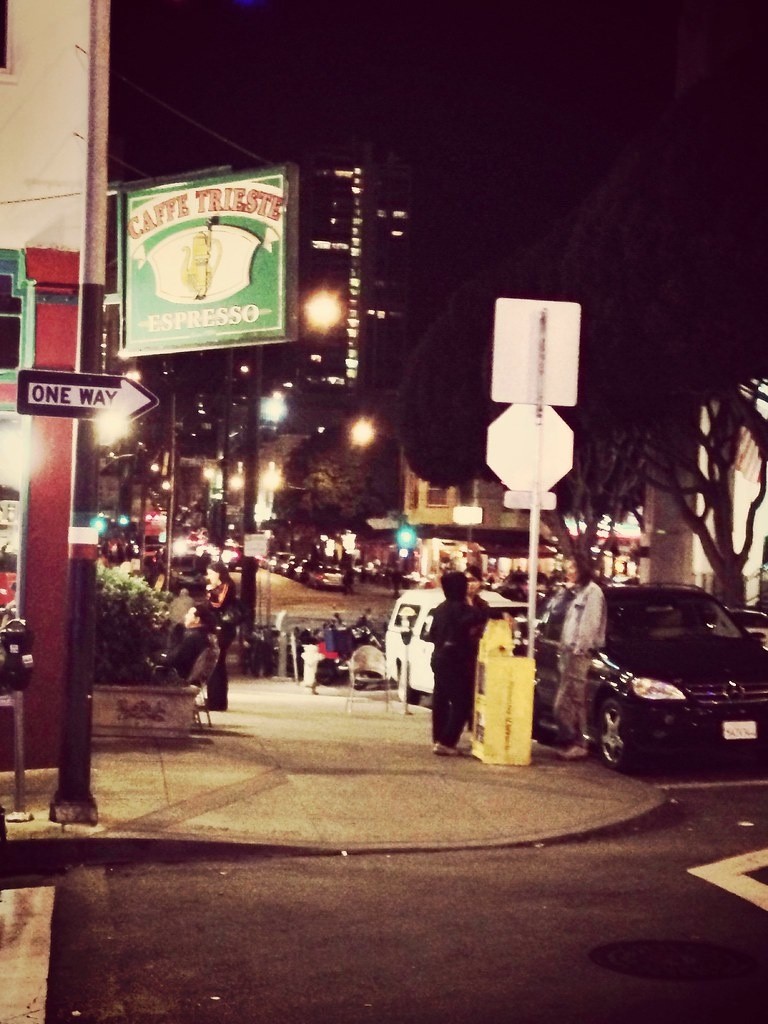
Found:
[244,604,386,688]
[352,559,394,582]
[255,552,345,593]
[724,604,768,650]
[161,555,207,594]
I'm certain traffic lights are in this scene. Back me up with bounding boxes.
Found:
[397,527,413,559]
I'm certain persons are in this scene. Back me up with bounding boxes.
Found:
[431,566,490,754]
[147,607,211,679]
[554,553,607,756]
[198,562,236,711]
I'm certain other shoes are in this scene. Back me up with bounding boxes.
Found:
[561,745,590,761]
[557,748,566,759]
[433,741,461,757]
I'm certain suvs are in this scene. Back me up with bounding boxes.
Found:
[534,582,768,779]
[385,589,529,707]
[0,550,19,608]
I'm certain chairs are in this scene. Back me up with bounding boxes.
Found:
[185,644,220,729]
[649,609,685,637]
[347,644,391,711]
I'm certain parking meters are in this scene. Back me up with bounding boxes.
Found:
[0,619,34,824]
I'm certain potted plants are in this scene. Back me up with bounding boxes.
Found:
[92,564,198,740]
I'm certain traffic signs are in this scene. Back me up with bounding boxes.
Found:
[18,369,157,429]
